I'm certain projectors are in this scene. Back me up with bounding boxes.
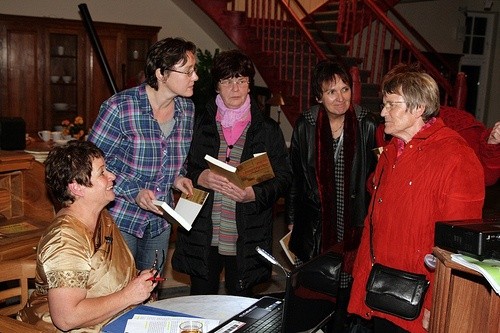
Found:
[434,217,500,262]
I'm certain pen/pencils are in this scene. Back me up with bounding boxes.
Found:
[149,278,168,280]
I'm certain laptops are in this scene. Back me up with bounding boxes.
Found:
[208,242,344,333]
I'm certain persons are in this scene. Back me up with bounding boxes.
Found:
[83,36,500,333]
[17,140,159,333]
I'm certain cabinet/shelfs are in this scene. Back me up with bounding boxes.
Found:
[429,246,500,333]
[0,13,162,138]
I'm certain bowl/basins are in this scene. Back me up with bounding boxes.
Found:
[58,139,68,146]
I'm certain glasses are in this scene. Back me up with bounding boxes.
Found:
[219,77,250,87]
[380,101,406,112]
[165,66,198,77]
[138,249,165,282]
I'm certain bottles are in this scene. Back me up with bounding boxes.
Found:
[133,47,138,60]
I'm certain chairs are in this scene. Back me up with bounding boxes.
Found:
[0,260,36,316]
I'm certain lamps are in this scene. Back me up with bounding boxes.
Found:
[268,88,285,124]
[255,245,290,277]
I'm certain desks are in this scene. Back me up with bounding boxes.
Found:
[100,294,412,333]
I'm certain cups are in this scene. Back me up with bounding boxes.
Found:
[179,320,203,333]
[38,130,50,142]
[63,76,72,83]
[54,103,68,110]
[58,46,65,55]
[51,132,63,141]
[51,76,60,83]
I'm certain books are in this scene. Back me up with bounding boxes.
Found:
[151,186,210,231]
[205,152,275,189]
[279,231,296,264]
[450,253,500,296]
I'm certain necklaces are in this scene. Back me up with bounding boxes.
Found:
[333,120,344,132]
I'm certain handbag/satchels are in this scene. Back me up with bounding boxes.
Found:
[290,250,344,297]
[364,263,430,321]
